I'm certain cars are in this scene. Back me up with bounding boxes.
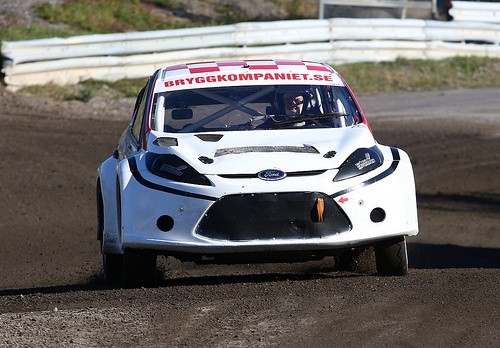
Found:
[96,57,419,283]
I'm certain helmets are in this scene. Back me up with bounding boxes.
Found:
[272,85,313,113]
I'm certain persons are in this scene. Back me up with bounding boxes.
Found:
[254,93,322,129]
